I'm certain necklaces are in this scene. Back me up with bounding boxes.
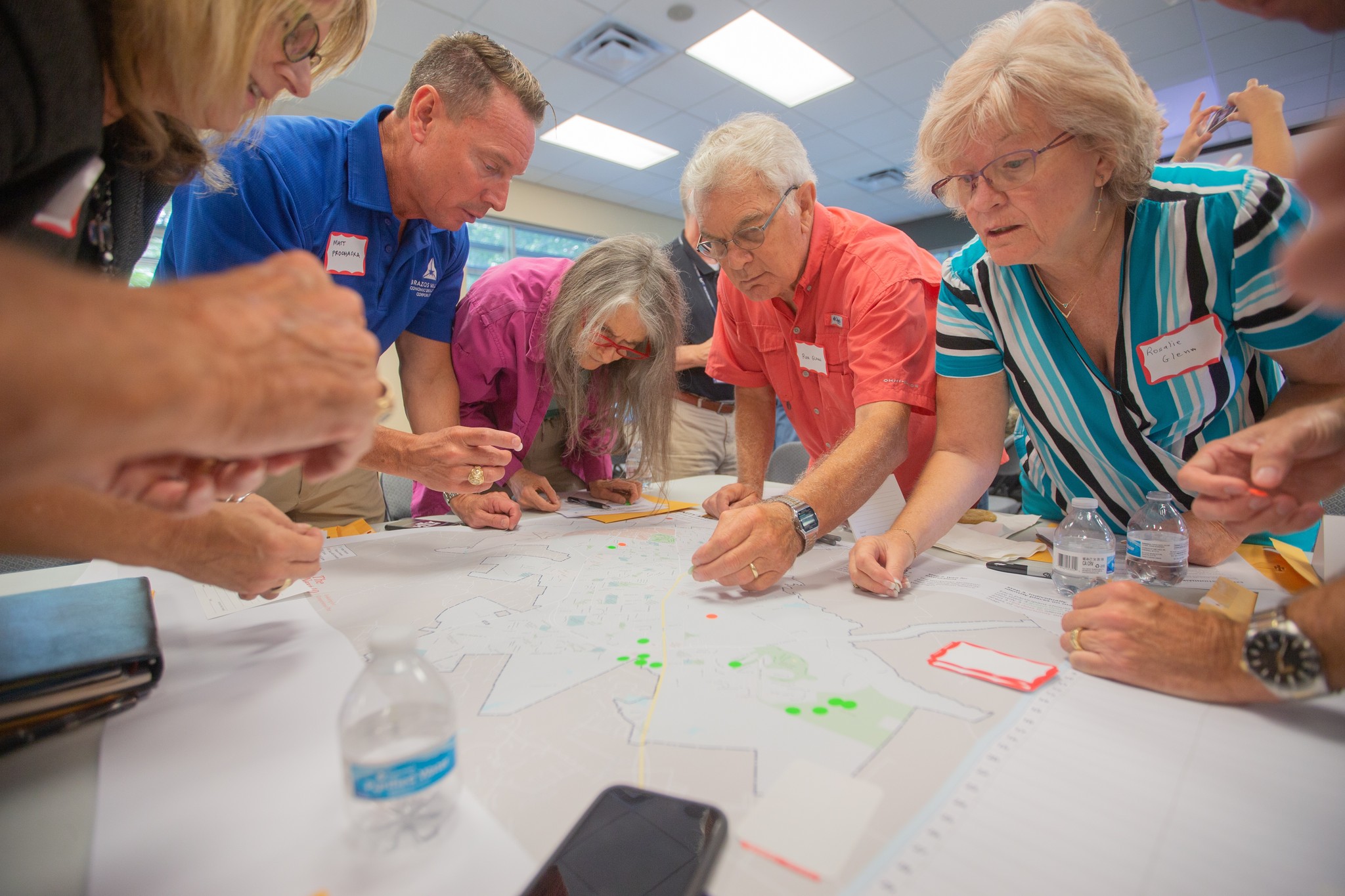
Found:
[1033,204,1120,319]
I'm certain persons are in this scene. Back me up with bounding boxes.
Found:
[649,178,737,483]
[0,0,376,603]
[413,234,689,521]
[850,1,1344,706]
[681,109,1011,593]
[154,31,558,532]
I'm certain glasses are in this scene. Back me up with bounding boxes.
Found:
[86,167,116,264]
[696,184,798,259]
[283,11,322,70]
[930,124,1079,209]
[581,305,652,360]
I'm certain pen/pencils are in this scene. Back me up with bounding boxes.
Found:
[987,560,1054,578]
[567,496,610,510]
[1036,532,1054,549]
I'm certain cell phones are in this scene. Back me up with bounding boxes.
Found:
[518,786,731,895]
[385,518,459,530]
[1207,105,1239,134]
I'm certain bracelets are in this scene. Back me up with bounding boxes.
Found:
[234,491,255,503]
[444,493,459,506]
[887,527,917,574]
[224,493,235,502]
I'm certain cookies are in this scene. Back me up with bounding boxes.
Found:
[957,509,997,524]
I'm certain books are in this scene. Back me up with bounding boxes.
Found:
[0,576,163,757]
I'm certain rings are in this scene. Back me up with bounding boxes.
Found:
[1070,628,1088,650]
[468,466,485,485]
[749,563,759,579]
[853,583,859,588]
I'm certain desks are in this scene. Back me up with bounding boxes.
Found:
[0,474,1345,896]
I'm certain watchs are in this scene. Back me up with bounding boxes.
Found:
[1237,600,1330,702]
[772,495,819,557]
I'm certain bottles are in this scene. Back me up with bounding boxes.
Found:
[1052,497,1118,597]
[341,626,461,865]
[1125,490,1189,586]
